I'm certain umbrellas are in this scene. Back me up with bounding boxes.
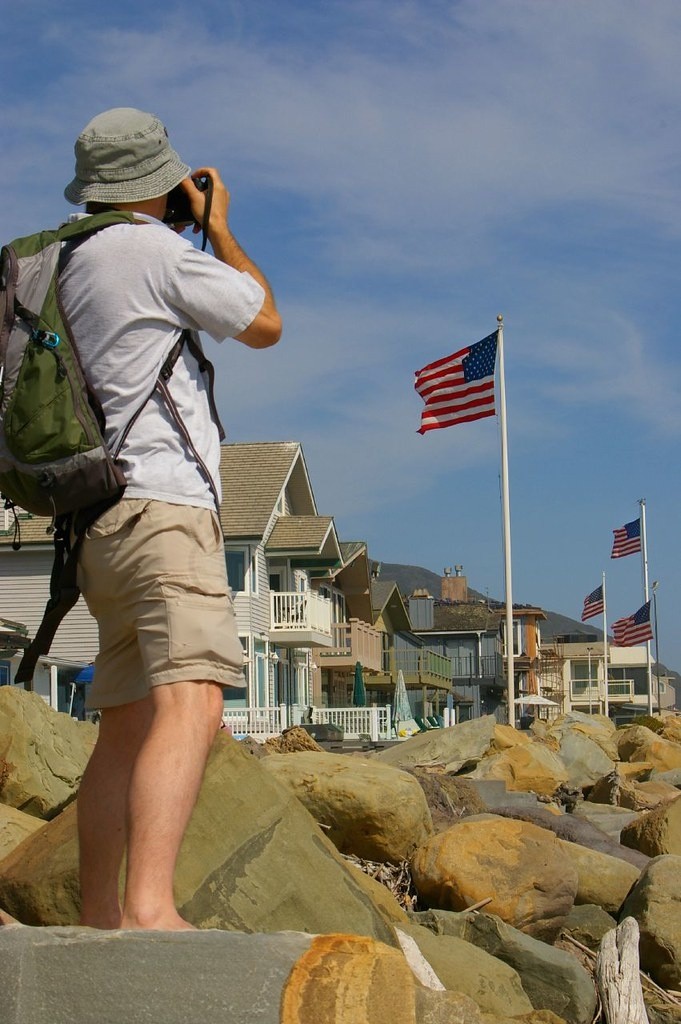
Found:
[352,661,366,708]
[514,693,559,720]
[390,670,413,722]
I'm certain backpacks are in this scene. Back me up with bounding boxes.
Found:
[0,212,189,519]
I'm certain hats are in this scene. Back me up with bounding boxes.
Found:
[63,107,191,206]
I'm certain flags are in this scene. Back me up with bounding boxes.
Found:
[611,518,642,559]
[414,329,497,435]
[611,600,654,647]
[581,585,603,621]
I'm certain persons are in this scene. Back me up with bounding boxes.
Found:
[60,109,284,934]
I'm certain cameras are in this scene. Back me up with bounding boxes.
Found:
[163,177,209,227]
